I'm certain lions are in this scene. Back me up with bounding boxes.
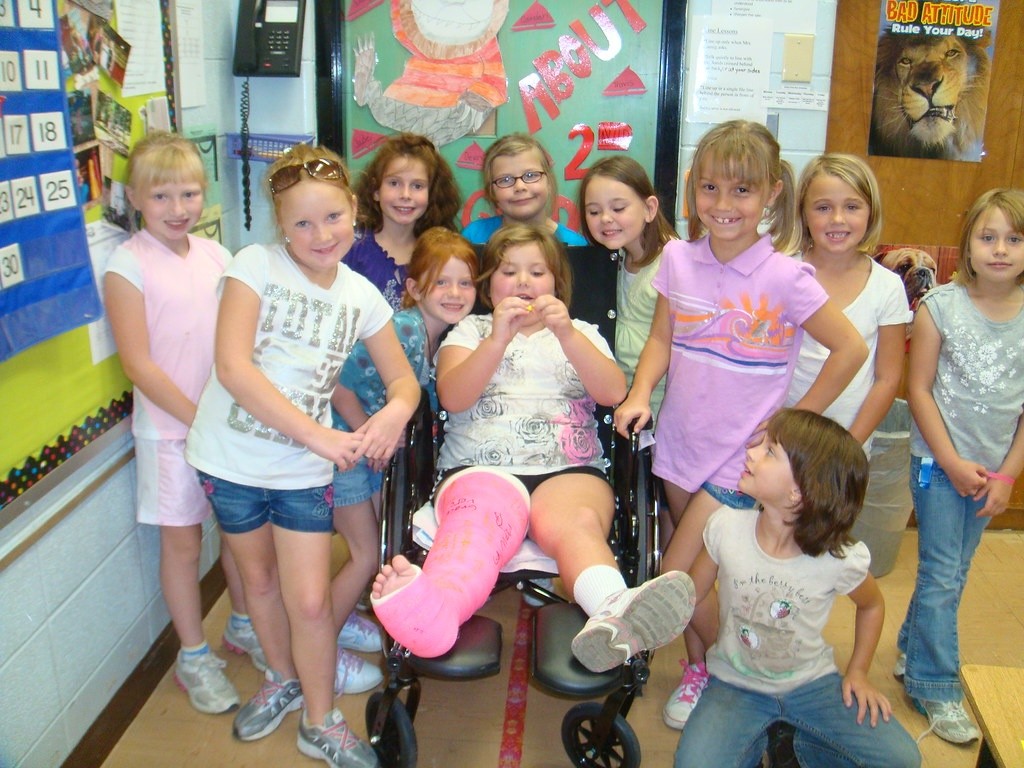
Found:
[867,1,991,162]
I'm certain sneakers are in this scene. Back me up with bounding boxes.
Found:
[913,696,978,745]
[894,654,907,678]
[298,708,379,768]
[222,617,267,672]
[571,571,698,673]
[172,648,239,715]
[333,644,385,696]
[662,659,708,731]
[232,670,303,741]
[339,610,384,652]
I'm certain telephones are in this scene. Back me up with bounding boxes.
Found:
[232,0,306,77]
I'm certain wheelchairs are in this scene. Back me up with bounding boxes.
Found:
[365,243,659,768]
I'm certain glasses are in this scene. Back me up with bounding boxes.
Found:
[492,171,546,188]
[269,159,348,200]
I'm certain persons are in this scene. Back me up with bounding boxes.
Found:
[180,146,422,768]
[101,130,272,716]
[309,118,1024,768]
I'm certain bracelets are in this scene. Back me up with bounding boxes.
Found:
[987,471,1015,486]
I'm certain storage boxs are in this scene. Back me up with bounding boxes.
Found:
[224,132,316,165]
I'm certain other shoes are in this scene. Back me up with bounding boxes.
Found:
[765,721,799,768]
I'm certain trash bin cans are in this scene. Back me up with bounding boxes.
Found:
[847,396,913,577]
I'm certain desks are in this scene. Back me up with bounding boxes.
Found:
[957,661,1024,768]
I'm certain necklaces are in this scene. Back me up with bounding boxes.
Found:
[423,318,433,366]
